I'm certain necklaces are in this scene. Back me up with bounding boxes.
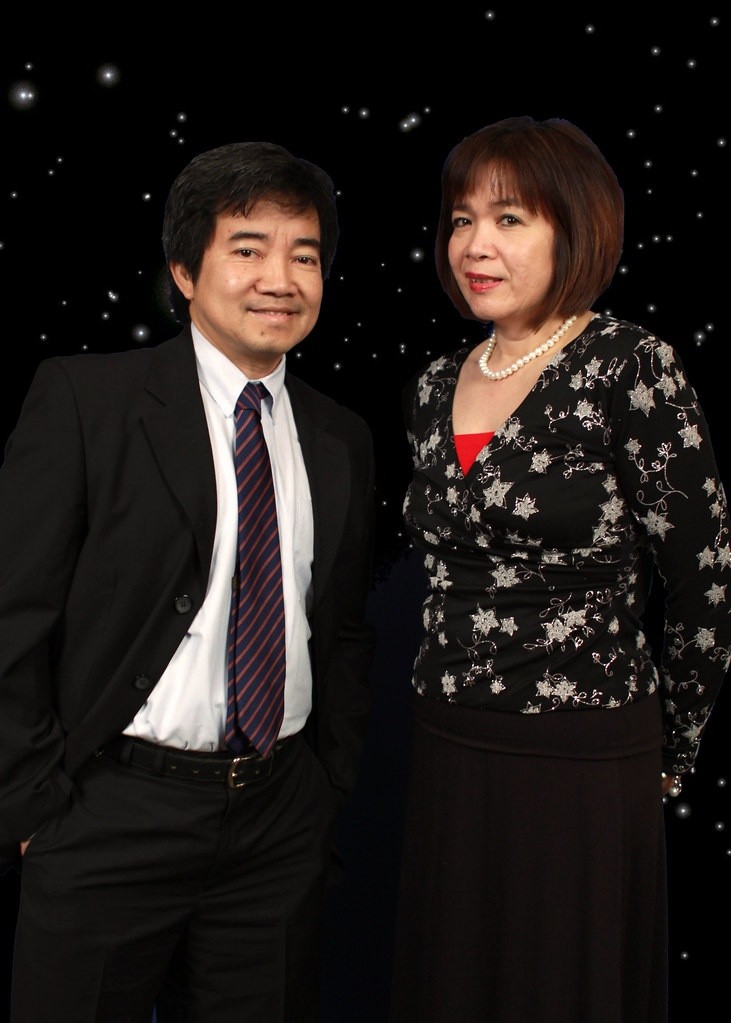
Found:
[478,313,576,380]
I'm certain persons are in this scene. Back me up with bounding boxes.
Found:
[401,115,730,1023]
[0,143,382,1023]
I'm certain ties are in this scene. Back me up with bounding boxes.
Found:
[231,377,288,756]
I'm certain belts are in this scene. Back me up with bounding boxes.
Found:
[119,741,274,790]
